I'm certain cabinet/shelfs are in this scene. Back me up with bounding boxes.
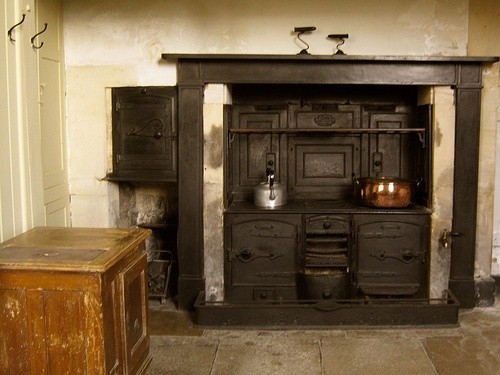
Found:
[0,1,73,245]
[224,102,434,302]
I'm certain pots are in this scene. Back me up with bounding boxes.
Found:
[350,172,424,207]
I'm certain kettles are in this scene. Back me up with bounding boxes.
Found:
[255,160,290,208]
